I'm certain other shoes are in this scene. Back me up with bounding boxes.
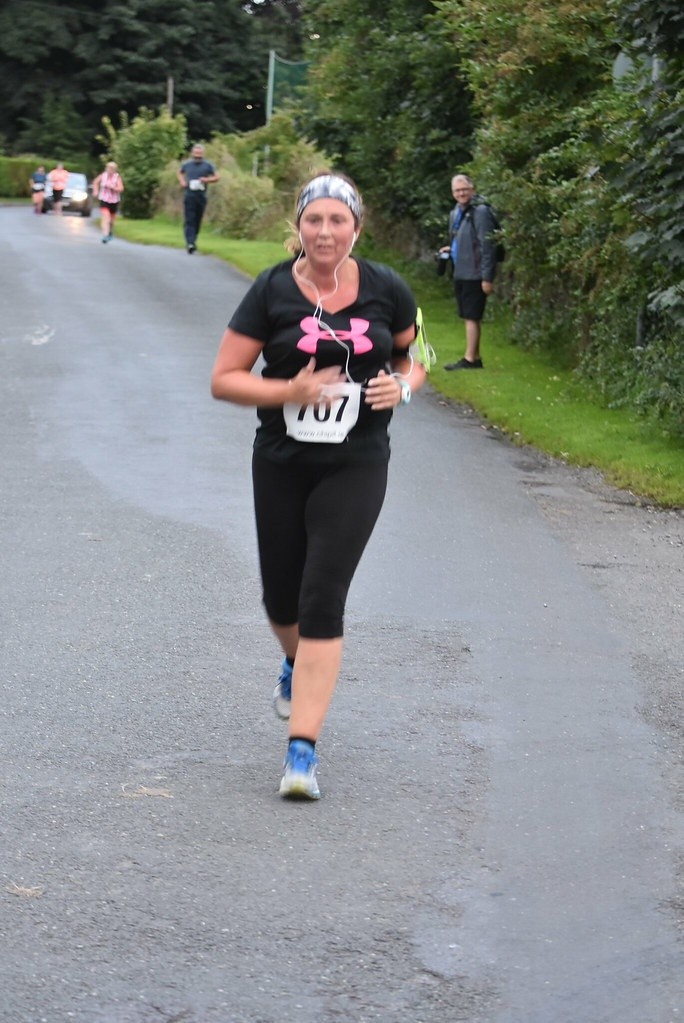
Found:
[102,233,112,244]
[187,243,197,254]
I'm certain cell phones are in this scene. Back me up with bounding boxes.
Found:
[415,307,431,373]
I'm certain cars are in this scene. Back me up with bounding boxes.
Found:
[41,172,95,215]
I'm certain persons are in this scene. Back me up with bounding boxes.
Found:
[29,166,47,215]
[176,143,218,254]
[438,174,498,371]
[92,159,125,244]
[49,162,69,216]
[209,171,437,803]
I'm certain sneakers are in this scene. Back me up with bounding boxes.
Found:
[279,739,320,800]
[273,656,293,721]
[443,356,482,371]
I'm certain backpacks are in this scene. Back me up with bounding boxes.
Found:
[470,201,506,263]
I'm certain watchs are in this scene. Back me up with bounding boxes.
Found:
[391,375,412,409]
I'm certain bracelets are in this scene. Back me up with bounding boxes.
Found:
[285,378,293,401]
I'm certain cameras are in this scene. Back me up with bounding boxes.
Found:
[434,252,450,276]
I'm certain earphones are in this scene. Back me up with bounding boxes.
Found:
[298,230,303,244]
[351,231,356,247]
